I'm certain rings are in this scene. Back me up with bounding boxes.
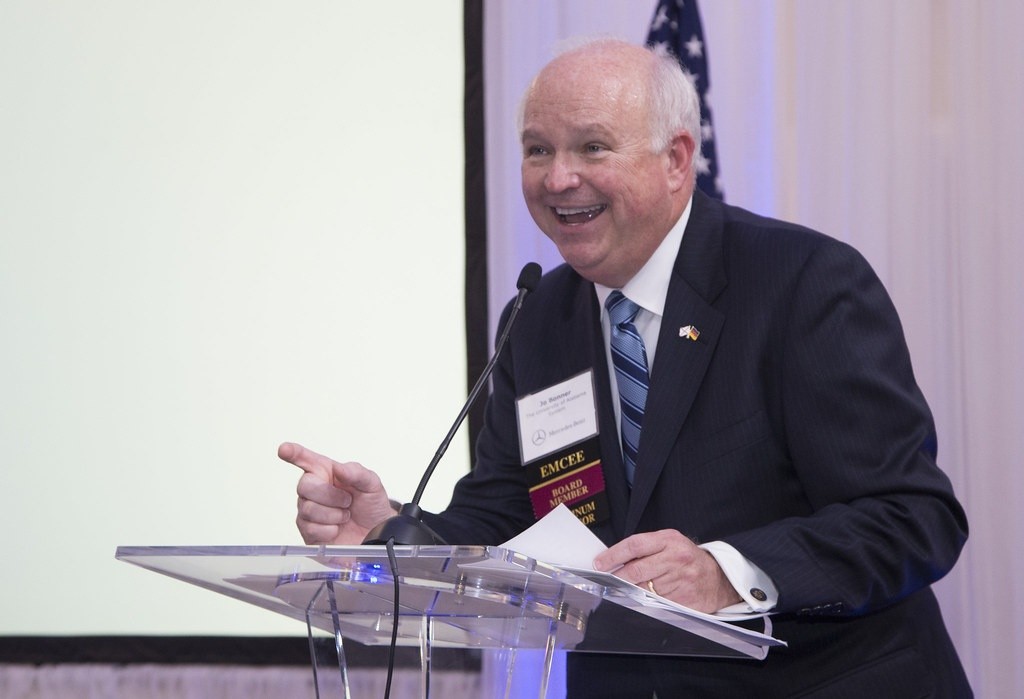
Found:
[648,580,657,594]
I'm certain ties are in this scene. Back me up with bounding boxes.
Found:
[603,291,655,492]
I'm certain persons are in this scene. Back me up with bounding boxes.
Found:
[278,34,976,698]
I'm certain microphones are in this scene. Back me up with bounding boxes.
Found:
[360,263,541,578]
